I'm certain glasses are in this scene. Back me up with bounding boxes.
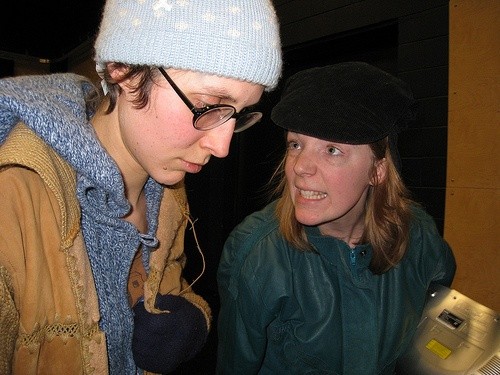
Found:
[156,67,265,132]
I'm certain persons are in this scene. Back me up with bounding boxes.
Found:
[214,60,457,375]
[0,0,282,375]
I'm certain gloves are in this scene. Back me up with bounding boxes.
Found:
[131,294,207,373]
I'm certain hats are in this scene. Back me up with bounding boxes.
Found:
[272,61,414,144]
[93,0,282,91]
